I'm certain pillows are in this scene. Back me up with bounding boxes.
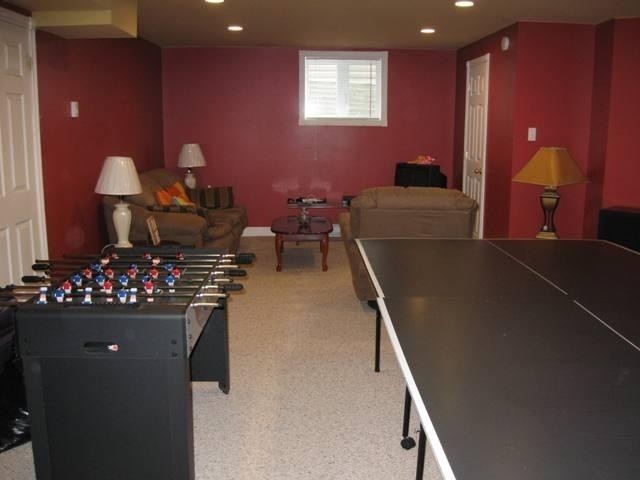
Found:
[155,182,189,206]
[173,196,199,215]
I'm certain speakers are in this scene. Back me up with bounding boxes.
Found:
[597,205,640,254]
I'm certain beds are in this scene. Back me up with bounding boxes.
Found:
[337,184,478,307]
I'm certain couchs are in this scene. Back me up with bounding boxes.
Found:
[101,168,249,250]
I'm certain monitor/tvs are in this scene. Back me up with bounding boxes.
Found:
[394,162,448,190]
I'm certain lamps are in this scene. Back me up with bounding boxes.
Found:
[94,157,144,249]
[511,147,592,240]
[177,143,207,189]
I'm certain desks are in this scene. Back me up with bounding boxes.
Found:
[354,237,640,480]
